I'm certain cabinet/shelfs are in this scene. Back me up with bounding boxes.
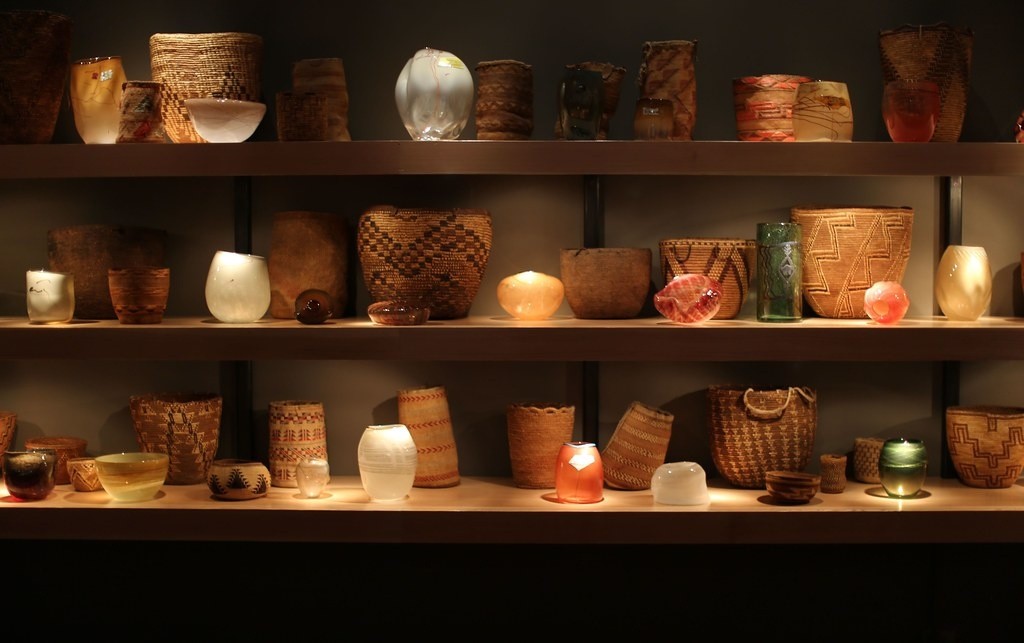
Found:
[0,141,1024,543]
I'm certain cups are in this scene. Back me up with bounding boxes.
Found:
[556,442,604,504]
[276,93,328,140]
[67,458,102,492]
[756,222,803,322]
[203,250,270,324]
[474,60,532,140]
[267,400,328,487]
[879,439,928,498]
[115,81,165,143]
[792,81,854,142]
[398,384,460,487]
[509,404,575,488]
[852,437,883,482]
[25,270,74,323]
[881,80,941,143]
[293,58,352,141]
[935,246,993,321]
[639,42,698,139]
[69,55,127,143]
[108,268,170,324]
[357,424,417,502]
[633,99,674,140]
[821,455,846,493]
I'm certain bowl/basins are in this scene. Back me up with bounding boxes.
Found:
[4,450,56,500]
[184,99,267,142]
[94,453,169,502]
[766,471,820,503]
[26,437,88,485]
